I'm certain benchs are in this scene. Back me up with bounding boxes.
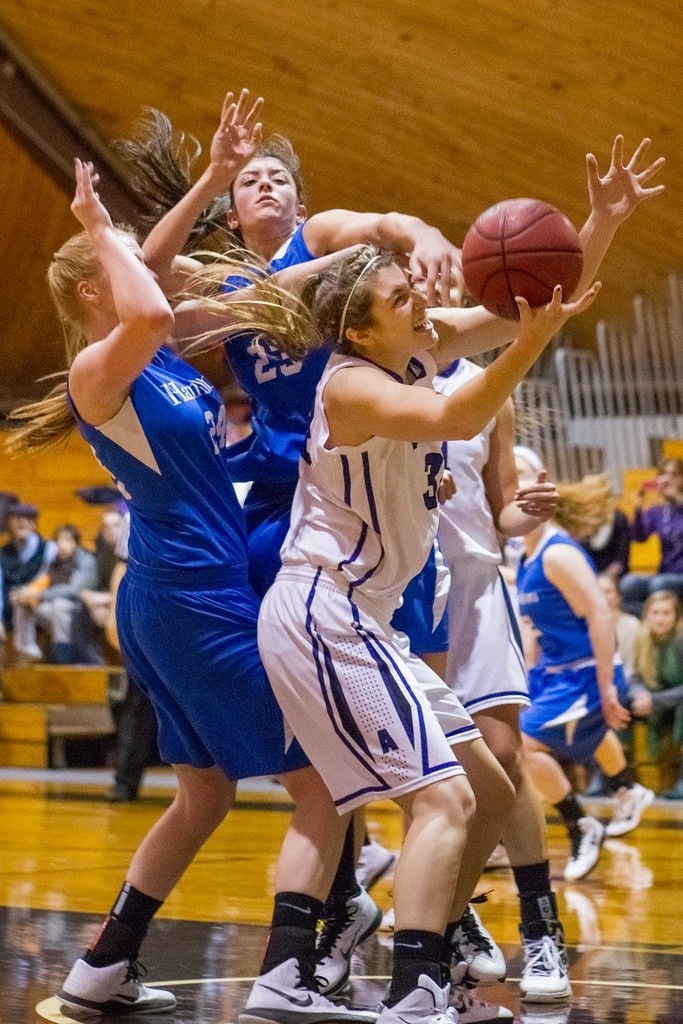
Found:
[1,431,125,768]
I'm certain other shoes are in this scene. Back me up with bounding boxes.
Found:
[585,773,606,795]
[664,779,683,798]
[111,780,134,800]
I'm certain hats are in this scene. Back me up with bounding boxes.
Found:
[9,504,36,518]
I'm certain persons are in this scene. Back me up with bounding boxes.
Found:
[0,87,682,1023]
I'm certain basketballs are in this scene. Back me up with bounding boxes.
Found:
[461,197,584,321]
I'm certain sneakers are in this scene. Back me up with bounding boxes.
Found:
[518,919,571,1001]
[483,843,509,868]
[606,781,655,837]
[236,957,382,1024]
[298,880,382,996]
[447,903,507,990]
[562,817,607,881]
[376,905,396,932]
[376,973,513,1024]
[56,956,178,1014]
[354,835,394,890]
